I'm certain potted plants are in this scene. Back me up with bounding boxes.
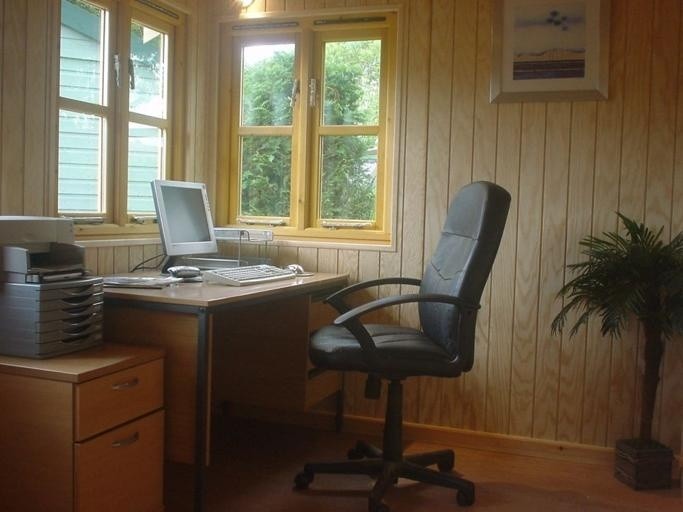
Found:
[549,210,683,493]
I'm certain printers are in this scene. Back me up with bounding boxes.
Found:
[0,215,92,283]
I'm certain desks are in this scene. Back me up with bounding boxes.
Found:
[101,269,350,511]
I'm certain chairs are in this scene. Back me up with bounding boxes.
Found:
[289,180,511,512]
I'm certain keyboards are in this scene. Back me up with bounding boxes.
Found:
[203,264,296,286]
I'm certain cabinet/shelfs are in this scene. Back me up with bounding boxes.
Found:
[0,341,172,512]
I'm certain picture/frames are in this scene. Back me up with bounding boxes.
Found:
[490,0,609,104]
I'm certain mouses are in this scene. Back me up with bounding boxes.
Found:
[286,264,305,275]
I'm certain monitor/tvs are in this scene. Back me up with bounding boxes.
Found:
[150,179,219,276]
